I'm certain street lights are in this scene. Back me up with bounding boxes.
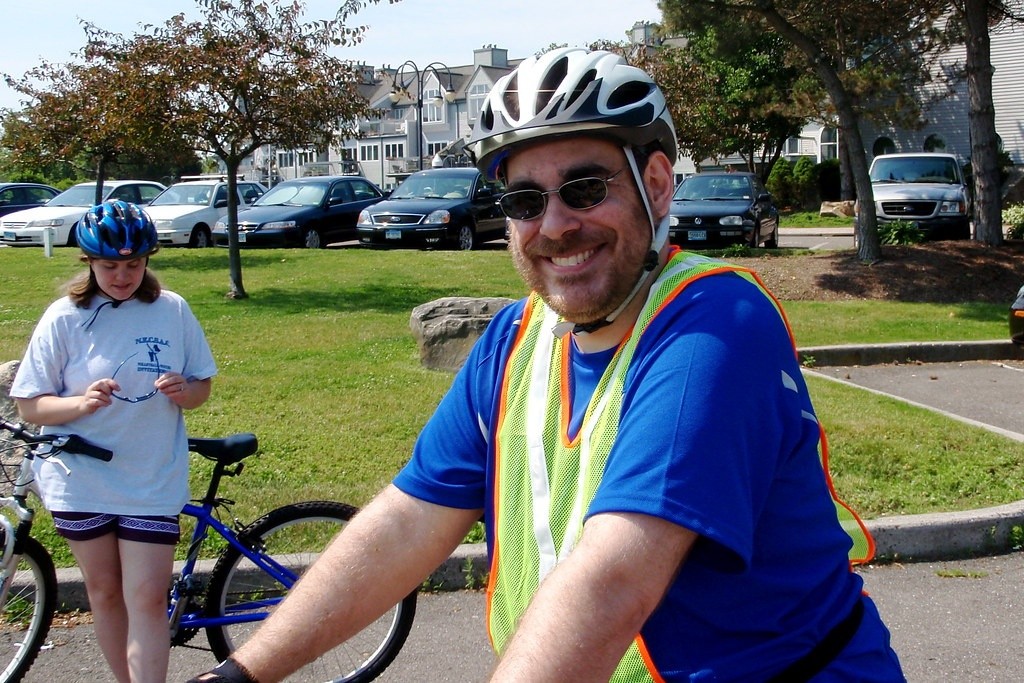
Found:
[389,59,457,172]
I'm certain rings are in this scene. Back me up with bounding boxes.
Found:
[180,383,183,391]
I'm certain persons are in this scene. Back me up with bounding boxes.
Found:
[186,46,910,682]
[7,200,215,683]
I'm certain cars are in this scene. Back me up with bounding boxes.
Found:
[670,170,781,249]
[144,173,271,249]
[0,183,64,217]
[0,178,193,247]
[210,176,389,249]
[354,166,511,252]
[853,152,973,240]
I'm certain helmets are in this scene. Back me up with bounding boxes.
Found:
[462,47,678,182]
[76,200,158,260]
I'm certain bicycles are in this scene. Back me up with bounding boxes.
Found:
[0,415,419,683]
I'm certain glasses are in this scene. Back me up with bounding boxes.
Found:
[111,343,159,403]
[494,161,630,221]
